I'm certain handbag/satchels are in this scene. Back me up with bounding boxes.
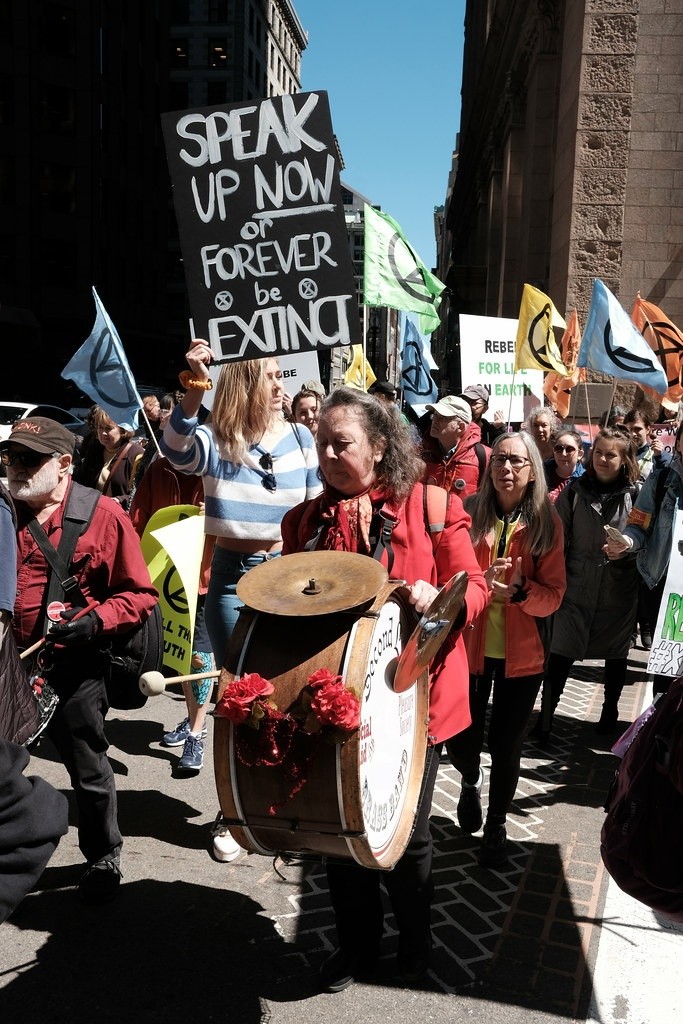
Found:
[600,676,683,924]
[107,604,164,709]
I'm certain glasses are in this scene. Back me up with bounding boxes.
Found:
[1,448,53,468]
[259,453,277,492]
[554,445,578,453]
[490,454,531,468]
[430,413,455,422]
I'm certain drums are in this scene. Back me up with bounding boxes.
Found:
[209,585,434,874]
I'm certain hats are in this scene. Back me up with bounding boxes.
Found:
[426,394,473,424]
[368,378,397,395]
[303,379,325,396]
[459,386,489,402]
[0,416,75,460]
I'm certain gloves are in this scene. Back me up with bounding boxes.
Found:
[44,606,103,653]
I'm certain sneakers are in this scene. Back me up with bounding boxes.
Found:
[162,718,208,746]
[79,859,123,905]
[212,825,240,861]
[177,736,203,771]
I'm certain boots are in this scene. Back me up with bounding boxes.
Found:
[532,662,570,747]
[595,658,626,736]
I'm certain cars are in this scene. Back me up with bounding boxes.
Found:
[0,402,174,493]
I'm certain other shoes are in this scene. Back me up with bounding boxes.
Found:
[397,934,433,981]
[478,825,511,872]
[320,949,366,991]
[628,622,652,650]
[457,767,485,833]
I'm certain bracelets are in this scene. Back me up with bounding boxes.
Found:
[179,370,213,389]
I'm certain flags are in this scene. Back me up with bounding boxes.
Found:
[542,303,584,420]
[514,285,567,376]
[363,203,446,333]
[575,278,668,395]
[632,294,683,415]
[345,344,377,392]
[401,319,439,418]
[61,289,144,432]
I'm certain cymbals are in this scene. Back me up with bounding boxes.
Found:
[233,545,392,619]
[392,569,471,695]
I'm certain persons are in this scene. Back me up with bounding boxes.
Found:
[0,416,160,925]
[529,405,683,747]
[72,339,567,992]
